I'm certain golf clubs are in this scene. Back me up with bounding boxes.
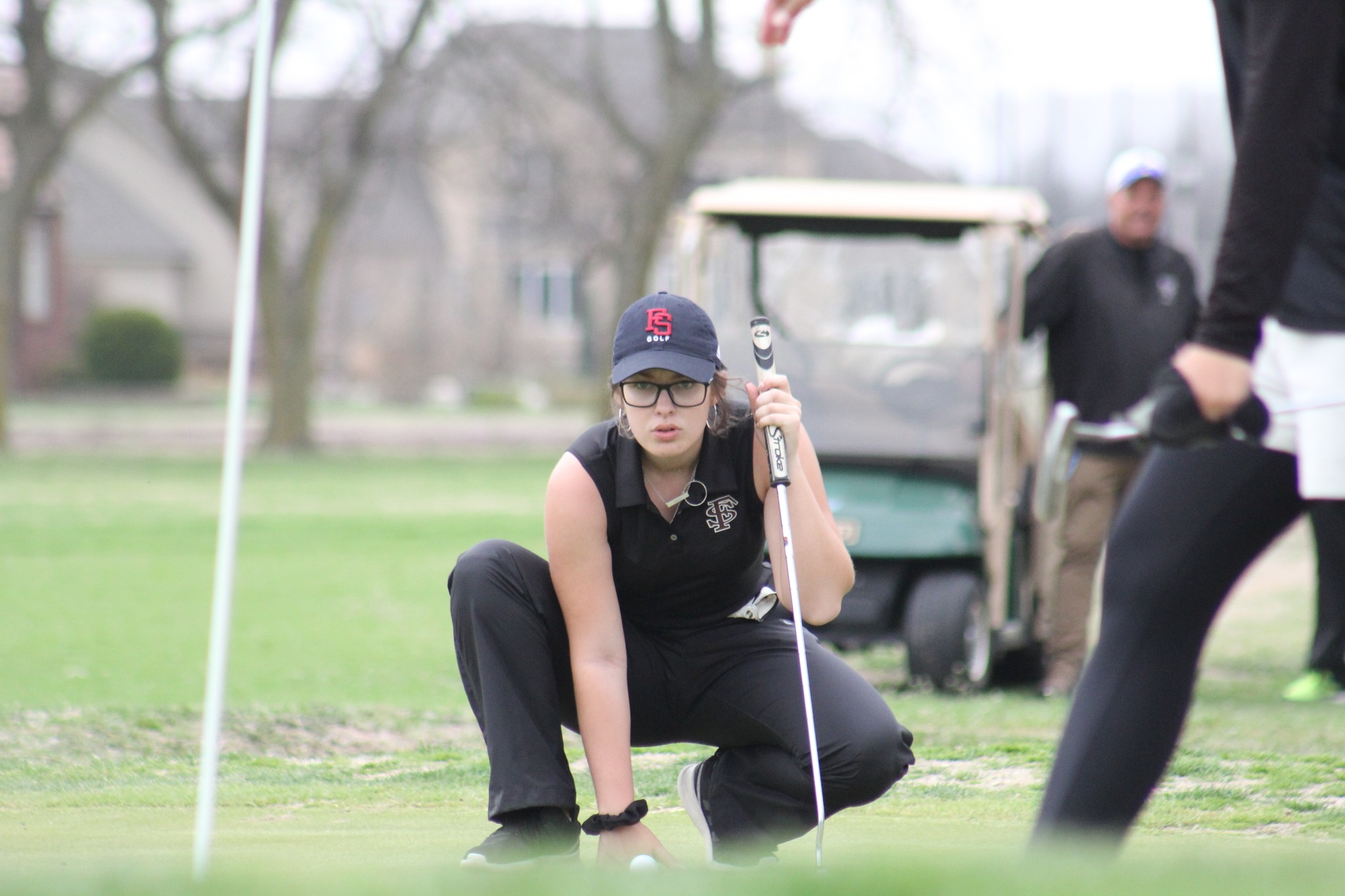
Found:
[745,310,833,881]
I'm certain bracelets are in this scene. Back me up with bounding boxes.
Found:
[583,799,649,835]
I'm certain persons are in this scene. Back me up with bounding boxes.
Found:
[446,293,916,870]
[1050,0,1345,856]
[1027,150,1345,701]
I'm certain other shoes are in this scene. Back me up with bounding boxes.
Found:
[1281,670,1338,701]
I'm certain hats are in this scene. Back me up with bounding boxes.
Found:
[1109,154,1166,192]
[611,291,721,384]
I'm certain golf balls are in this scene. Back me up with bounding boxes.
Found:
[628,855,657,874]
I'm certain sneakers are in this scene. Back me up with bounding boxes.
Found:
[461,819,581,868]
[676,756,780,872]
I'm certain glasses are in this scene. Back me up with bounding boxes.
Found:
[619,381,709,408]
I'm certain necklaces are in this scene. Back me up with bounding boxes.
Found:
[636,450,708,507]
